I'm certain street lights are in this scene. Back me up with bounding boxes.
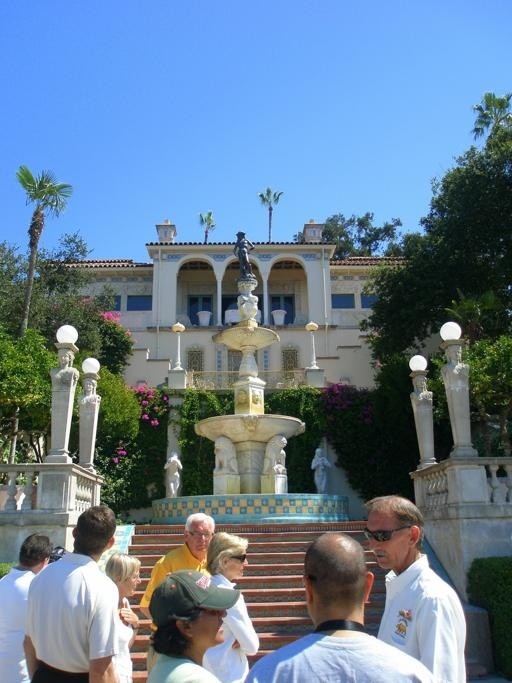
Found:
[408,353,440,470]
[438,320,478,457]
[45,324,81,462]
[171,320,186,370]
[79,356,102,466]
[304,320,320,369]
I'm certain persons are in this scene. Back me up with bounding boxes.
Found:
[202,529,260,683]
[310,447,332,494]
[163,452,183,497]
[409,375,434,399]
[0,505,140,683]
[138,513,215,674]
[49,348,80,387]
[142,569,241,683]
[233,231,257,281]
[441,345,470,389]
[363,496,468,682]
[244,532,436,682]
[77,377,102,405]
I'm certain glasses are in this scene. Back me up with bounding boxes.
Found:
[188,531,213,538]
[363,526,411,542]
[230,555,246,562]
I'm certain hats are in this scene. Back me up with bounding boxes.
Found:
[148,569,240,629]
